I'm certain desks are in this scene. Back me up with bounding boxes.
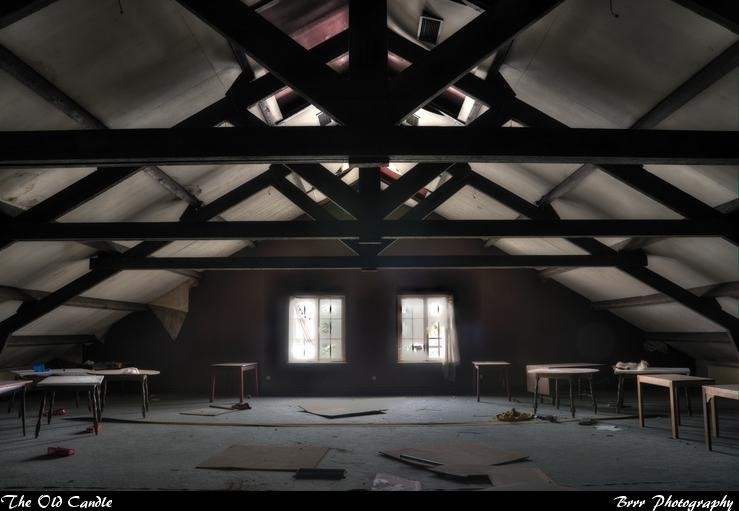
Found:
[0,363,160,438]
[472,359,739,450]
[209,361,259,404]
